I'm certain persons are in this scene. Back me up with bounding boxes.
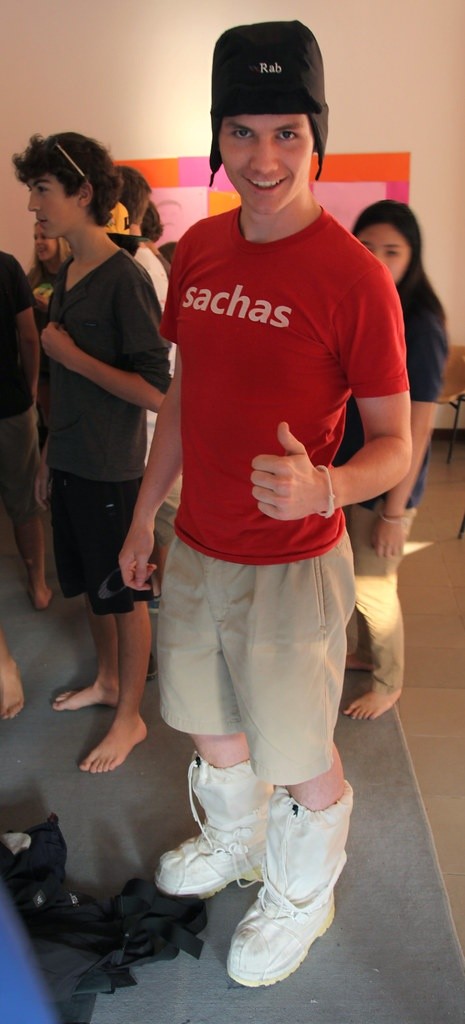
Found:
[109,165,182,612]
[331,199,447,720]
[0,251,52,609]
[118,21,412,987]
[0,630,58,1024]
[13,133,171,772]
[27,220,69,447]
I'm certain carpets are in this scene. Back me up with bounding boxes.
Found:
[0,494,465,1024]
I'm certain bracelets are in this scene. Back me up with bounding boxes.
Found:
[378,502,404,523]
[316,466,334,518]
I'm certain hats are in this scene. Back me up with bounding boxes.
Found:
[209,22,328,187]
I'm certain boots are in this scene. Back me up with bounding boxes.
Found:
[155,748,275,899]
[226,776,354,987]
